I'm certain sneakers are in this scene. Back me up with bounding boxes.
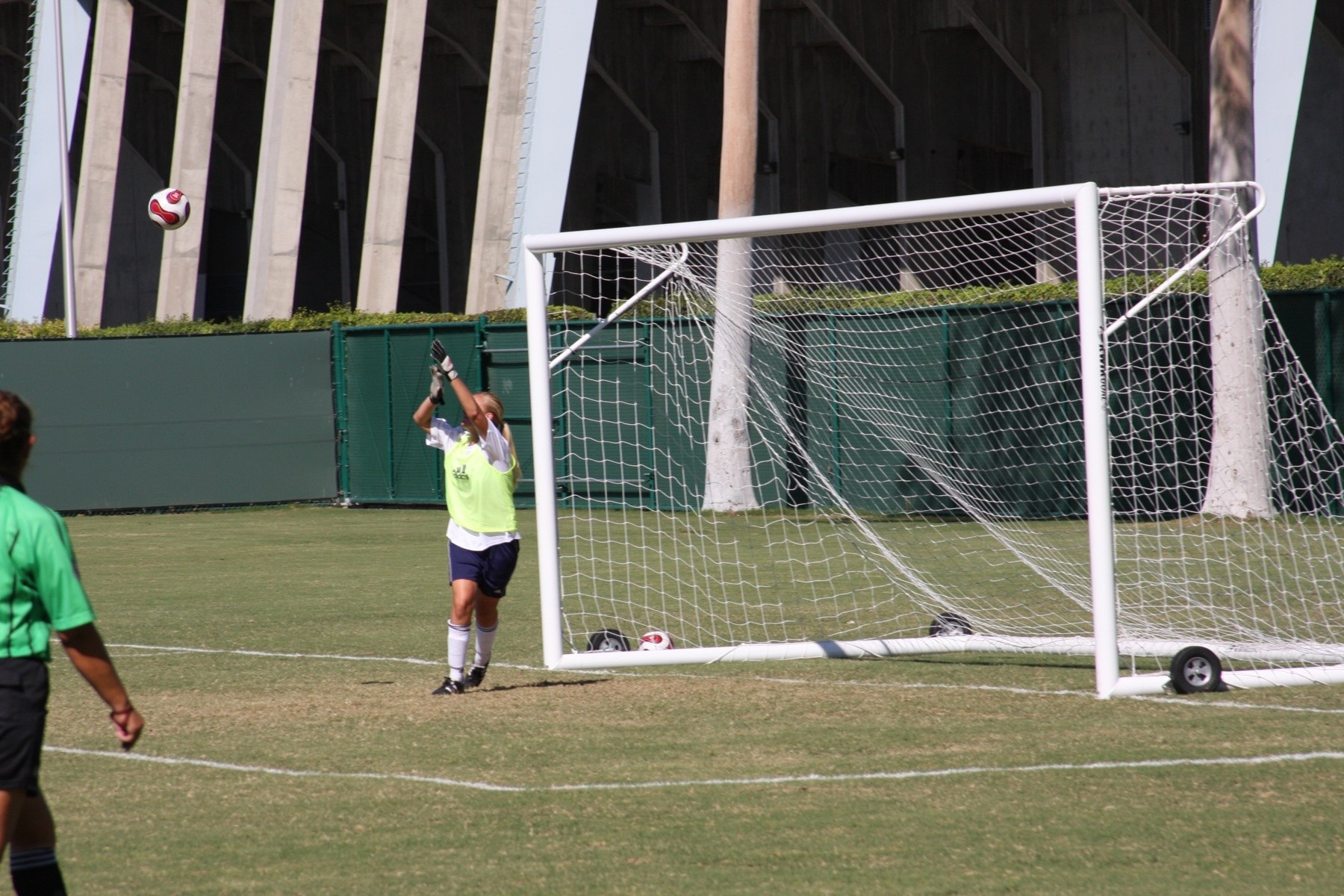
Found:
[465,654,490,688]
[432,676,466,695]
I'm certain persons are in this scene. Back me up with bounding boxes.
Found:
[0,388,144,896]
[412,338,522,695]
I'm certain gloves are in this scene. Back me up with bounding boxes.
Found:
[430,339,458,382]
[429,364,446,406]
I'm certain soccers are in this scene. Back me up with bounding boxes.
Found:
[639,630,673,652]
[146,188,190,230]
